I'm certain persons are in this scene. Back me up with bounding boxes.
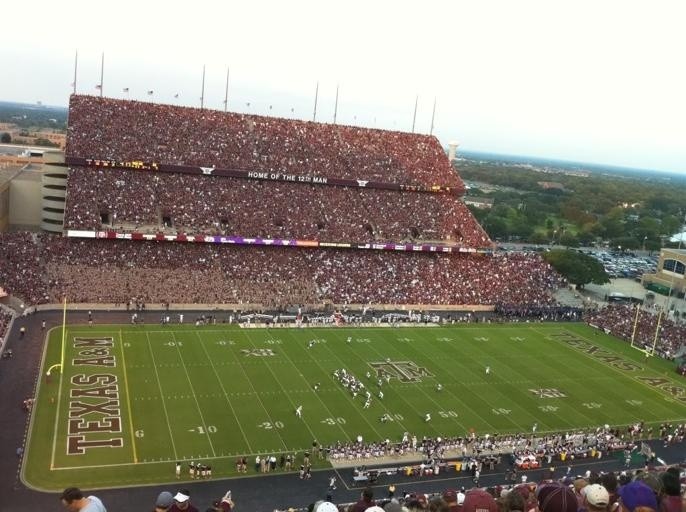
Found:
[23,396,36,413]
[144,416,686,512]
[581,299,686,378]
[2,349,14,361]
[295,405,303,420]
[314,381,320,392]
[0,94,601,357]
[59,486,108,511]
[436,382,443,391]
[423,411,431,422]
[485,363,491,375]
[331,358,394,415]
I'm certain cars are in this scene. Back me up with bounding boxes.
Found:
[495,244,602,256]
[601,248,660,281]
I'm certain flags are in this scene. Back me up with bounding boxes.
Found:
[94,85,102,91]
[70,82,76,88]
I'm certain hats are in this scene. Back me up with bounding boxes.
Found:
[535,482,579,512]
[615,481,659,511]
[461,489,498,512]
[579,483,610,509]
